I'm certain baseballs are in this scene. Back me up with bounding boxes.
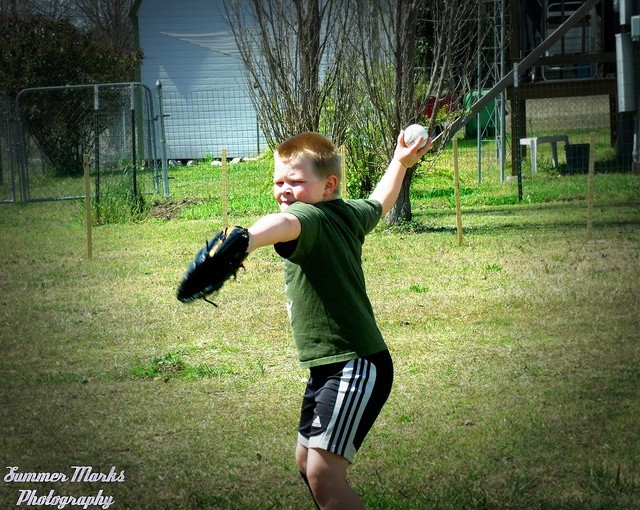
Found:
[404,124,429,149]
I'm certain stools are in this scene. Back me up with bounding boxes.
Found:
[520,134,569,176]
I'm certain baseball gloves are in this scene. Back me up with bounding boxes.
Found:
[177,225,249,306]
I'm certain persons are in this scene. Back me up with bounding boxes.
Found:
[177,129,432,510]
